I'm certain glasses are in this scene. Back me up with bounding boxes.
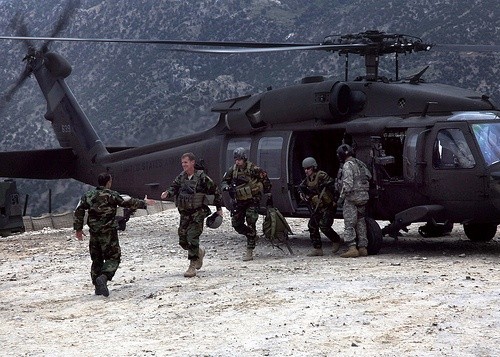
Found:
[304,166,313,170]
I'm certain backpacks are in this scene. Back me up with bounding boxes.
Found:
[263,207,295,244]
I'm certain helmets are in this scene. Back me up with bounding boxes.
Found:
[233,146,248,160]
[336,143,353,159]
[206,212,222,229]
[302,156,318,168]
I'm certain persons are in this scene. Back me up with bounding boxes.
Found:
[74,172,156,297]
[336,145,372,258]
[222,147,273,261]
[160,152,223,278]
[297,157,344,257]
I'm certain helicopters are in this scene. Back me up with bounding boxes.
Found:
[0,0,500,255]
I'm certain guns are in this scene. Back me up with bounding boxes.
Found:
[296,184,319,226]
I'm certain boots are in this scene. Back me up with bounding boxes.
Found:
[340,245,368,258]
[95,273,110,297]
[331,237,343,254]
[242,235,261,261]
[306,247,324,256]
[184,246,205,277]
